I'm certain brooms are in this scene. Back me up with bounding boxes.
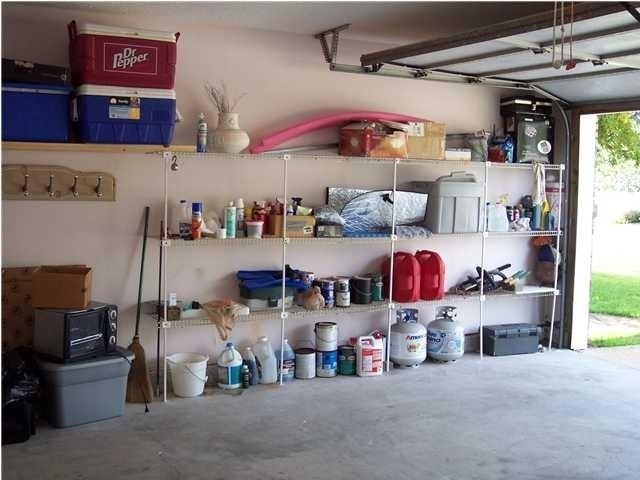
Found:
[125,206,155,403]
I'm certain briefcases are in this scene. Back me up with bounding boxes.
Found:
[2,58,68,84]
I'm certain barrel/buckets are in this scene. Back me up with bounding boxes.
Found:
[414,250,446,301]
[168,196,193,237]
[273,337,295,384]
[384,251,421,303]
[253,336,276,384]
[349,275,376,304]
[166,352,210,397]
[355,333,384,378]
[487,202,508,232]
[241,346,259,385]
[313,321,338,352]
[216,342,244,391]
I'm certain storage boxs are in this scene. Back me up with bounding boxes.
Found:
[239,285,296,311]
[66,18,181,90]
[1,82,75,143]
[500,94,553,116]
[339,121,393,157]
[411,179,485,234]
[268,214,315,237]
[479,322,544,356]
[30,264,92,311]
[70,82,184,146]
[35,343,135,430]
[404,119,446,160]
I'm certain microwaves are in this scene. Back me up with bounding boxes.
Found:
[34,301,118,362]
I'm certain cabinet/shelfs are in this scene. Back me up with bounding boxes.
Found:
[137,151,565,404]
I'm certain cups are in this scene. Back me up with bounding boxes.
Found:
[244,220,264,238]
[216,228,227,240]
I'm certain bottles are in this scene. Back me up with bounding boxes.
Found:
[506,202,555,231]
[224,199,245,238]
[242,365,249,387]
[196,112,208,152]
[191,201,203,240]
[241,345,258,387]
[275,338,294,381]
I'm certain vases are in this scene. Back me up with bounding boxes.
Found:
[205,112,250,154]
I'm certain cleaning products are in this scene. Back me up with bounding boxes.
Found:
[224,196,246,238]
